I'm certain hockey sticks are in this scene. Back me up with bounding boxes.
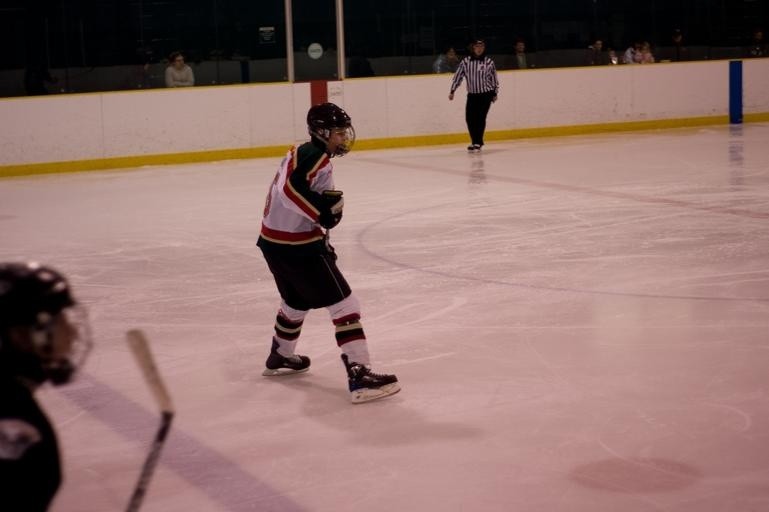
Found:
[124,328,175,512]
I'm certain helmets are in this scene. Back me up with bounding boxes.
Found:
[0,262,75,314]
[307,102,351,127]
[469,39,488,55]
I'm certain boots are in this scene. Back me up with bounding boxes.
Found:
[265,352,311,370]
[468,143,484,150]
[347,362,399,392]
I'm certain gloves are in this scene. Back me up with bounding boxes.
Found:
[322,196,343,228]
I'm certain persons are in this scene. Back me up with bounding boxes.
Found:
[431,42,461,74]
[0,261,97,512]
[582,39,657,69]
[749,26,769,58]
[667,26,689,63]
[163,52,196,87]
[254,101,401,395]
[448,35,500,152]
[514,40,536,69]
